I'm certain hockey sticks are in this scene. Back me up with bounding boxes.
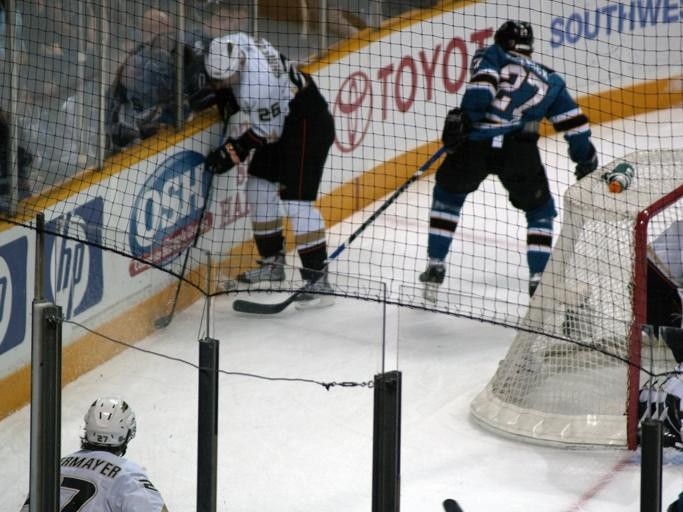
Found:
[153,105,233,328]
[233,147,447,314]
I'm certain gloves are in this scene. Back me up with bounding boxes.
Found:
[575,157,597,179]
[208,148,234,175]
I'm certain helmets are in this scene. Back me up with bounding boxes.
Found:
[84,398,136,448]
[204,37,240,80]
[494,18,533,53]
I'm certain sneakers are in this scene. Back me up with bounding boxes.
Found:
[419,265,444,282]
[237,264,284,283]
[295,283,333,301]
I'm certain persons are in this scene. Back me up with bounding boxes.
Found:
[1,1,369,207]
[634,220,681,447]
[20,395,167,511]
[204,33,335,308]
[418,19,599,297]
